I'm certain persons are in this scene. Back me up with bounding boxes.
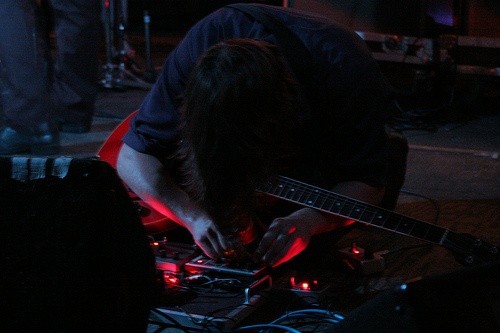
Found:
[116,4,409,263]
[0,0,103,155]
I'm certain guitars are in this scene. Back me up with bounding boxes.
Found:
[97,108,496,267]
